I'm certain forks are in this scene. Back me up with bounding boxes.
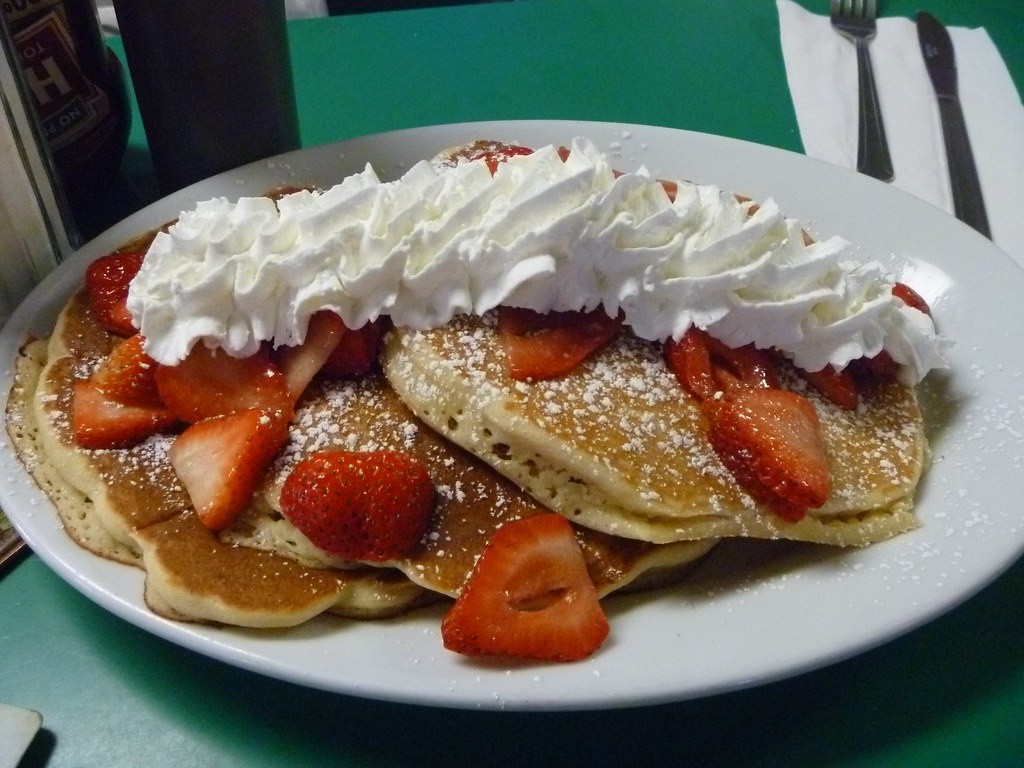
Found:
[830,0,894,182]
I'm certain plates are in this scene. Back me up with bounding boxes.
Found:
[0,119,1024,711]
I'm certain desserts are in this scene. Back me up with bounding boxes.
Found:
[5,145,955,662]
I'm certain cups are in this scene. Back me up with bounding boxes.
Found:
[112,0,303,194]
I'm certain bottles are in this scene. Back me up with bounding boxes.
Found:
[0,0,133,232]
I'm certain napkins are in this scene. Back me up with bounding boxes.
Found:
[775,0,1024,269]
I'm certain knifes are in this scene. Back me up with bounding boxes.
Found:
[915,11,992,241]
